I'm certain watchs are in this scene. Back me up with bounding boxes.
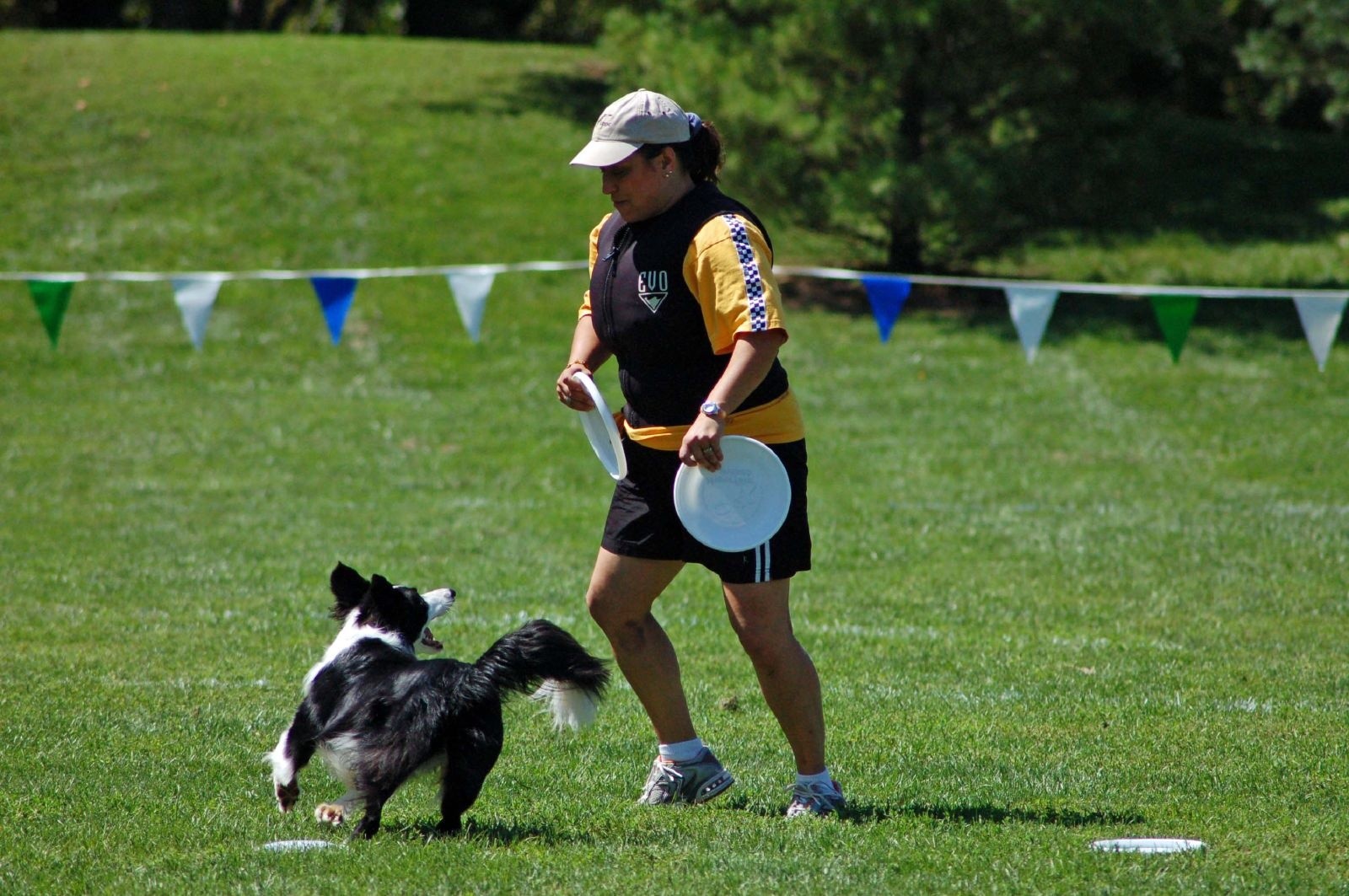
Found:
[701,402,727,419]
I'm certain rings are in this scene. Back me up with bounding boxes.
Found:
[566,397,574,407]
[703,447,712,453]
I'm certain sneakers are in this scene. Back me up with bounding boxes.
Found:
[782,780,849,818]
[637,747,734,805]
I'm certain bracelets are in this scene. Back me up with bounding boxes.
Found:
[566,360,589,370]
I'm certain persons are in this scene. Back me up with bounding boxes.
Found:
[558,88,848,818]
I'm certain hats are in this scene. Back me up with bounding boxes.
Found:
[569,88,691,166]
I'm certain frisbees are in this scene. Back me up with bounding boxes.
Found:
[1088,838,1209,854]
[671,432,792,554]
[571,370,628,482]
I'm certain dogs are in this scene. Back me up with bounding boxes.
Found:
[260,559,616,844]
